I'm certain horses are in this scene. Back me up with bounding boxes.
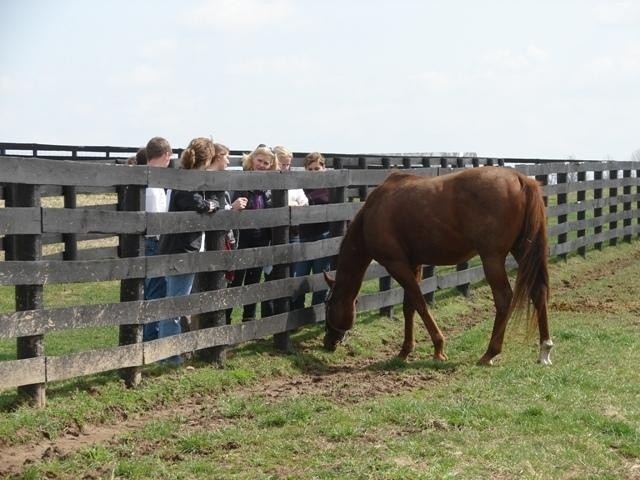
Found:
[321,166,554,367]
